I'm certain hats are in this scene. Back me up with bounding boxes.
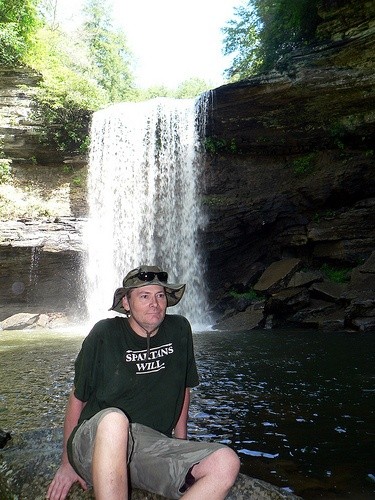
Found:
[108,265,186,315]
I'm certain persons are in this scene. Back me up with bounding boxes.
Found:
[45,265,241,500]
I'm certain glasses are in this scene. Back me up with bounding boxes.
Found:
[125,272,168,282]
